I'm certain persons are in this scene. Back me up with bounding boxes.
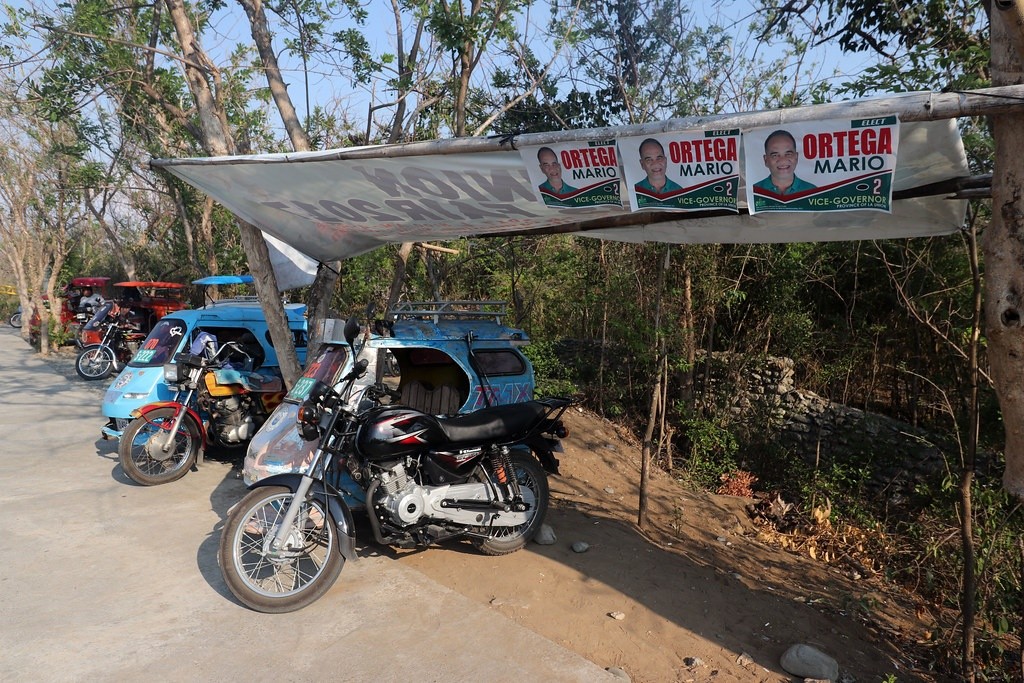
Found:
[78,286,105,307]
[753,130,818,195]
[635,138,686,194]
[537,147,577,195]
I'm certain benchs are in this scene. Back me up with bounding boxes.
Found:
[400,382,459,418]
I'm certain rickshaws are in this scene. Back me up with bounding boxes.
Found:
[9,274,113,347]
[103,295,317,486]
[74,281,193,381]
[218,316,576,614]
